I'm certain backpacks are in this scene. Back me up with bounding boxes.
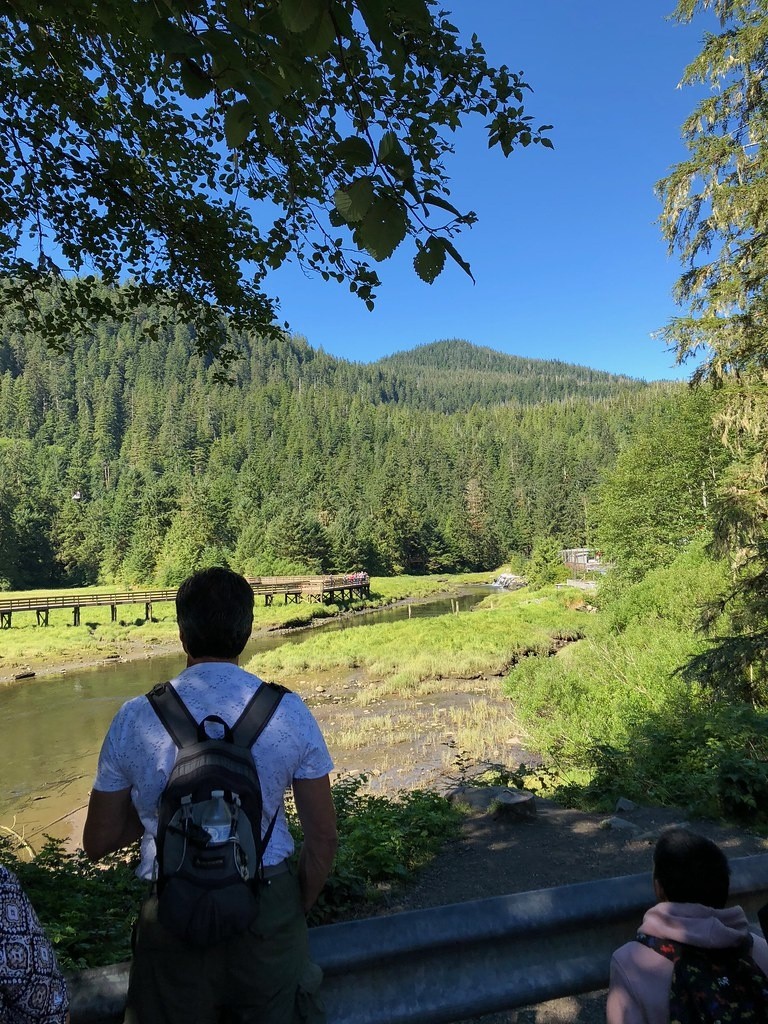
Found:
[144,681,292,947]
[630,932,768,1024]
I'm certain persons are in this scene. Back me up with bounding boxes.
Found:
[329,571,369,587]
[82,565,341,1024]
[0,862,71,1024]
[606,827,768,1024]
